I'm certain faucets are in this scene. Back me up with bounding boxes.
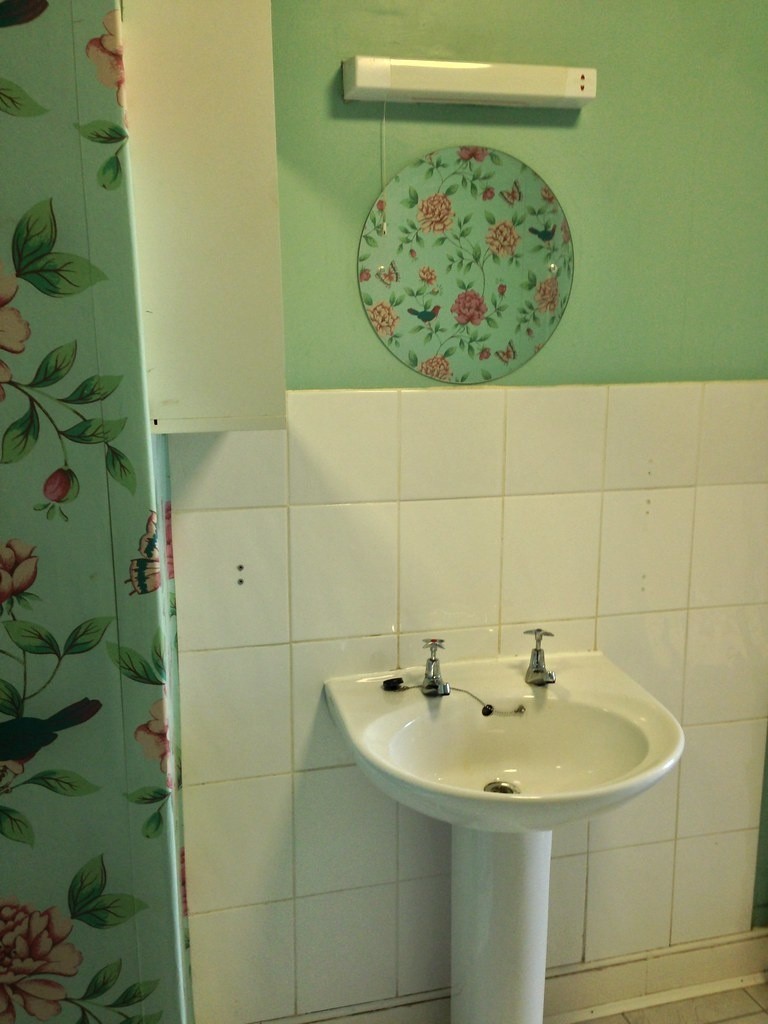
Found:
[421,638,451,696]
[523,628,557,686]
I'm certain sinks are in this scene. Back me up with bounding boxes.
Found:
[323,650,684,832]
